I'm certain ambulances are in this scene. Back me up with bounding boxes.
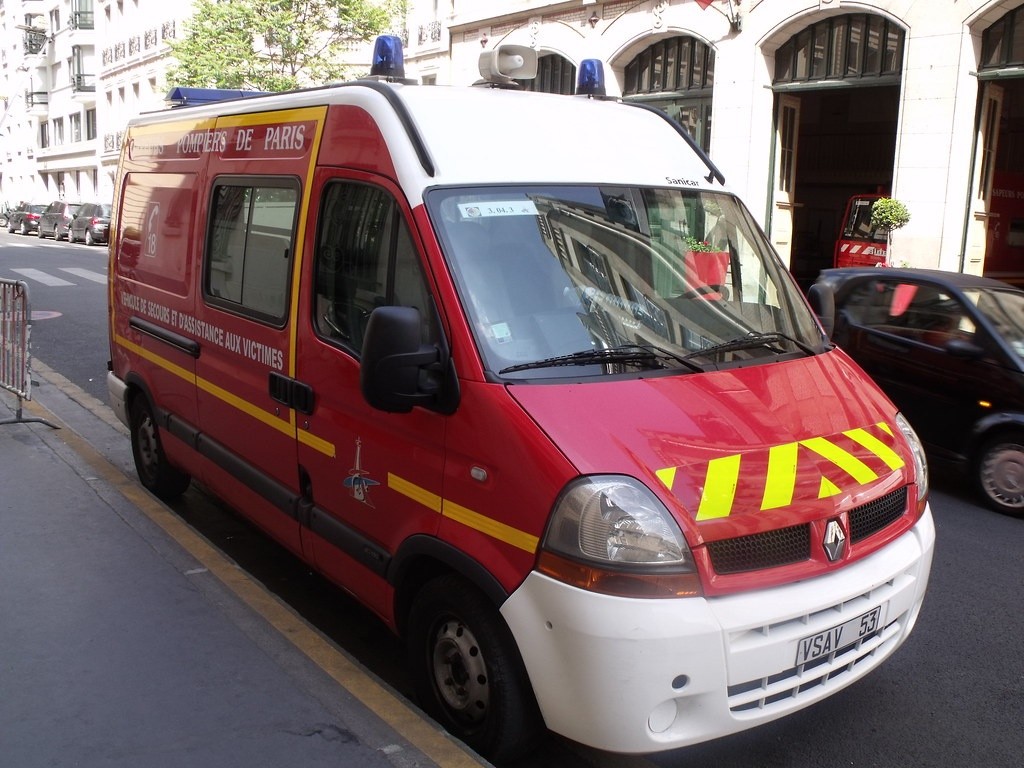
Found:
[833,181,1024,288]
[102,31,938,754]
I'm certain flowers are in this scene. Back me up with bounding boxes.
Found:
[682,236,723,253]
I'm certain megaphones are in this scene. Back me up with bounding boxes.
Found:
[478,45,538,83]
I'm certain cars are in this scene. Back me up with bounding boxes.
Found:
[66,201,113,247]
[812,266,1024,515]
[0,202,51,235]
[37,200,84,240]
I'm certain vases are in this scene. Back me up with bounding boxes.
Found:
[683,251,729,300]
